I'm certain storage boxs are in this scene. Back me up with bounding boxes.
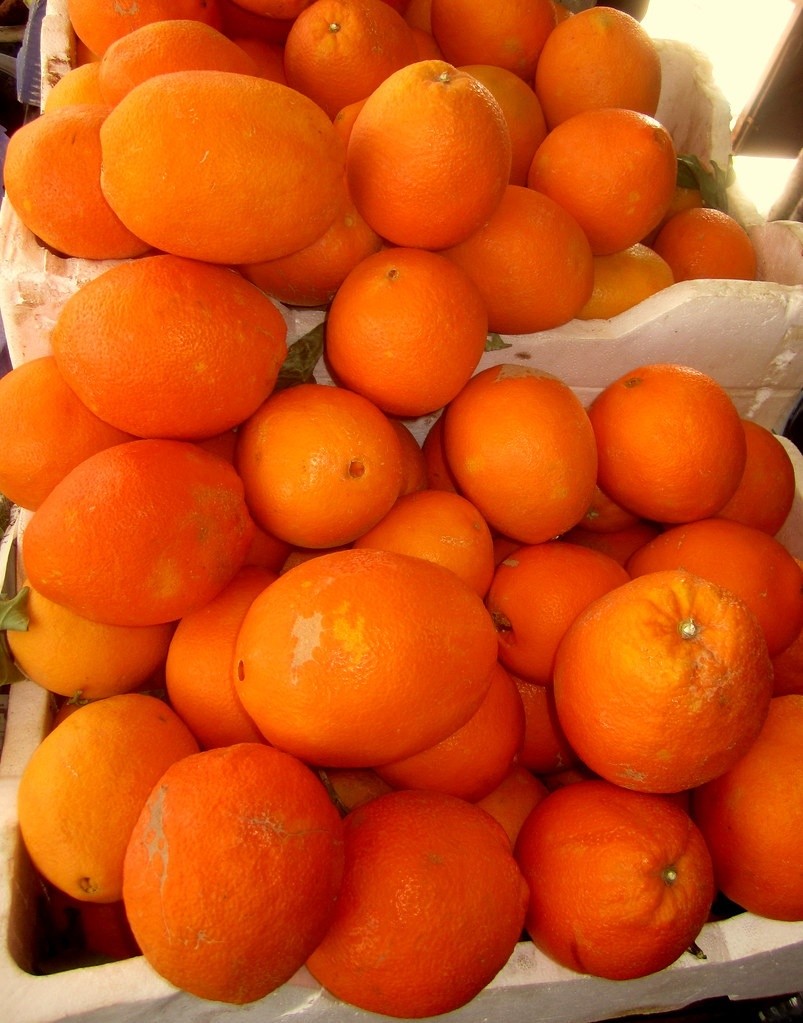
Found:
[0,0,803,1023]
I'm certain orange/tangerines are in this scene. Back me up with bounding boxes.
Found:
[0,0,803,1021]
